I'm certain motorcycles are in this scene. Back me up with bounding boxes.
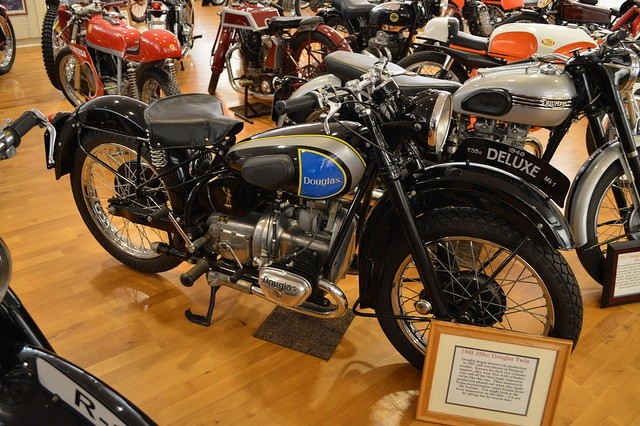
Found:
[207,0,353,117]
[394,6,639,166]
[148,0,202,88]
[0,5,16,76]
[54,2,183,121]
[316,0,497,79]
[123,0,180,23]
[493,0,621,35]
[0,109,158,426]
[43,55,583,388]
[271,27,640,290]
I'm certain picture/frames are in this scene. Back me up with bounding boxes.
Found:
[601,238,640,306]
[415,320,574,425]
